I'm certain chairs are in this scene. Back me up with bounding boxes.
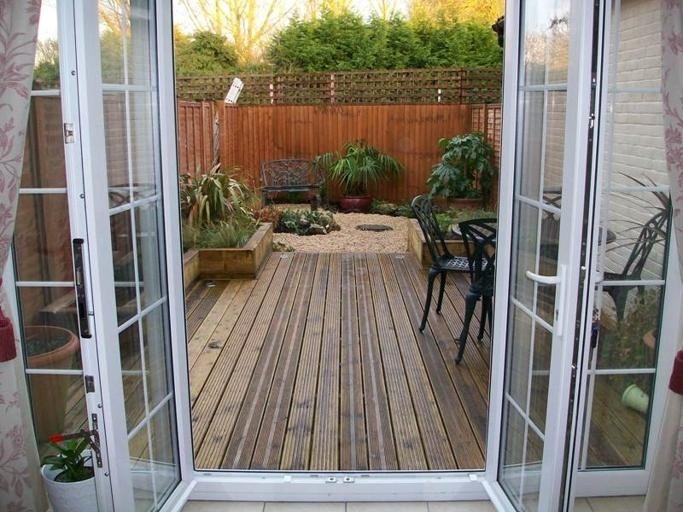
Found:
[595,209,668,322]
[540,188,564,224]
[455,218,496,364]
[410,194,496,331]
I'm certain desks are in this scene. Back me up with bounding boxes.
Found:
[448,221,616,339]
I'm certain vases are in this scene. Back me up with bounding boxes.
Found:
[22,325,81,444]
[642,326,658,365]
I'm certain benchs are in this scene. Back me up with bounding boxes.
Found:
[257,159,325,211]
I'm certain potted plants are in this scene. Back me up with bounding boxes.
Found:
[424,131,498,210]
[40,435,100,512]
[315,141,405,212]
